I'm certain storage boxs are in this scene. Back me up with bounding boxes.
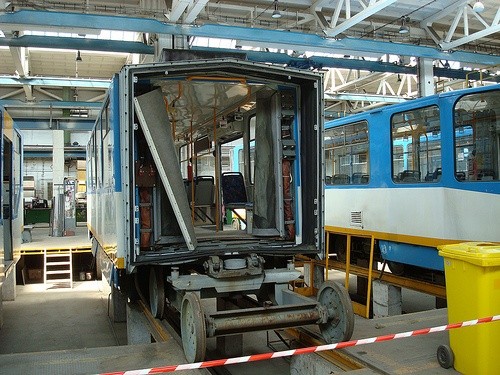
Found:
[28,267,41,280]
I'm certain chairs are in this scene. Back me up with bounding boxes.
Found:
[456,170,496,182]
[392,167,441,183]
[325,172,368,184]
[186,171,254,230]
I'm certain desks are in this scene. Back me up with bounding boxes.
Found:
[24,224,35,241]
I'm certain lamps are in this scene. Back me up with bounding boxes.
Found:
[271,0,281,19]
[398,16,409,35]
[445,60,450,68]
[73,88,78,98]
[76,49,83,62]
[473,0,485,12]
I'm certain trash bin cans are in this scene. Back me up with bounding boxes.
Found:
[437,241,500,375]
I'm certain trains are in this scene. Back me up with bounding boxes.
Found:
[83,58,354,363]
[0,105,24,279]
[229,84,500,275]
